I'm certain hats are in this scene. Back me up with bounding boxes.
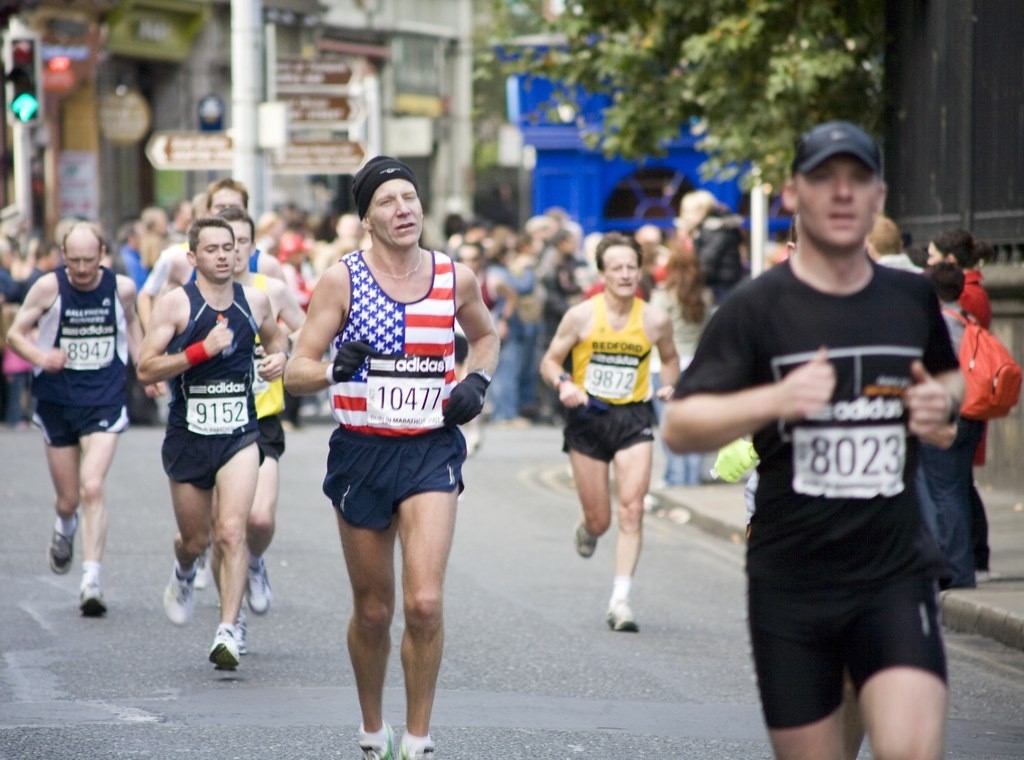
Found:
[351,156,419,221]
[790,120,883,176]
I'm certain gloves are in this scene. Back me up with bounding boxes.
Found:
[325,339,381,385]
[442,368,493,427]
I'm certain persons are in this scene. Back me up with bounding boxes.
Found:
[0,190,752,486]
[6,222,167,618]
[135,217,290,672]
[284,154,500,759]
[862,215,991,593]
[210,208,291,656]
[539,231,680,633]
[660,121,969,756]
[157,176,288,589]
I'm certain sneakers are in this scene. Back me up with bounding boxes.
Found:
[606,596,638,631]
[46,511,79,574]
[79,581,107,617]
[357,719,397,760]
[574,511,596,557]
[193,546,211,589]
[235,610,248,655]
[397,731,435,760]
[208,626,240,671]
[244,555,272,614]
[162,559,198,626]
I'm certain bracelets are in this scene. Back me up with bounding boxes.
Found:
[184,340,209,366]
[947,394,960,426]
[554,372,572,387]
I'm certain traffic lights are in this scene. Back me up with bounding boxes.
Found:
[6,32,43,129]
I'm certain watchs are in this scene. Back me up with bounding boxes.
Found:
[474,368,493,385]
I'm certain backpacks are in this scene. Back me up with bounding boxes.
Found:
[941,310,1024,420]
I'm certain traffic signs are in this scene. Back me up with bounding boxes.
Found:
[145,128,233,169]
[274,56,368,180]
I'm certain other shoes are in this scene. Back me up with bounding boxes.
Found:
[935,570,993,593]
